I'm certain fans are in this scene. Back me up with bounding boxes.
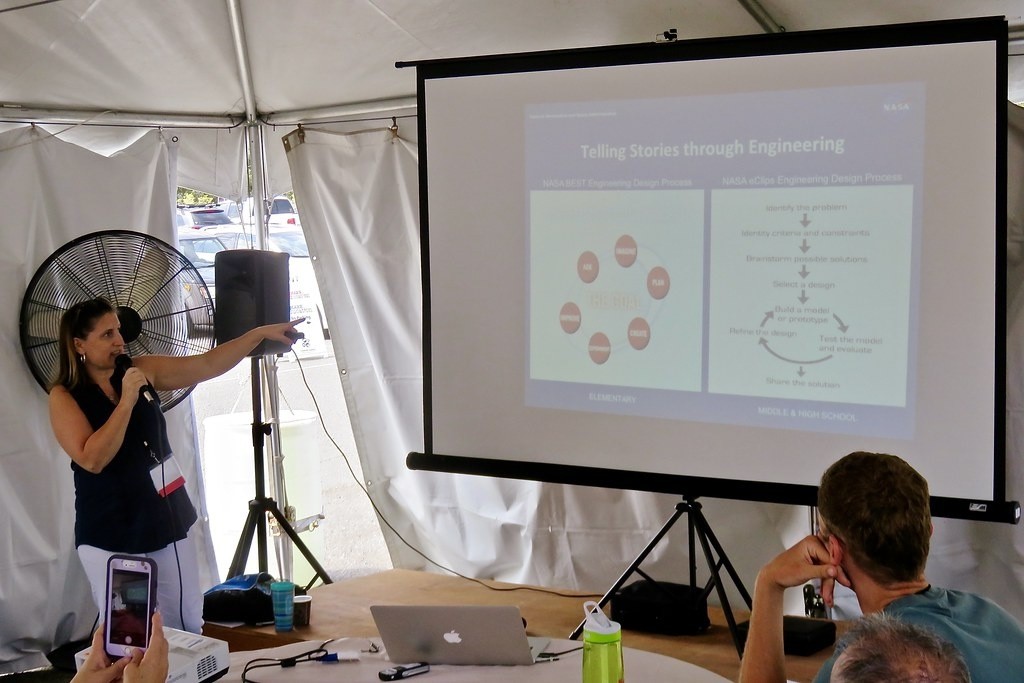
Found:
[18,229,217,413]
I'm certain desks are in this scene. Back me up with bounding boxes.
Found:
[200,568,853,683]
[215,635,731,683]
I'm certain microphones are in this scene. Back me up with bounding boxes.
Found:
[114,354,154,403]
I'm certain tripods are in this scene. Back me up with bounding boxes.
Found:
[226,358,333,597]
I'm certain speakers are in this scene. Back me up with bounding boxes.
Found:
[215,249,292,357]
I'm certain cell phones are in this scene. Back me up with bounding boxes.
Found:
[103,555,158,660]
[378,663,430,681]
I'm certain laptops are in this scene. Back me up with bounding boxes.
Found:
[371,606,551,666]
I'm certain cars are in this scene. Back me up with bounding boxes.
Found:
[177,195,330,340]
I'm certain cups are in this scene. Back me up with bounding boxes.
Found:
[293,596,312,627]
[271,582,295,631]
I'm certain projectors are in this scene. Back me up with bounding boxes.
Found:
[75,625,230,683]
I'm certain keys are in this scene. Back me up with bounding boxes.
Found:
[379,661,430,680]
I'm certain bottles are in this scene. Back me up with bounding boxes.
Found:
[581,601,624,683]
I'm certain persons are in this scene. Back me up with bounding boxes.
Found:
[49,297,306,636]
[830,610,972,683]
[69,612,169,683]
[738,451,1024,683]
